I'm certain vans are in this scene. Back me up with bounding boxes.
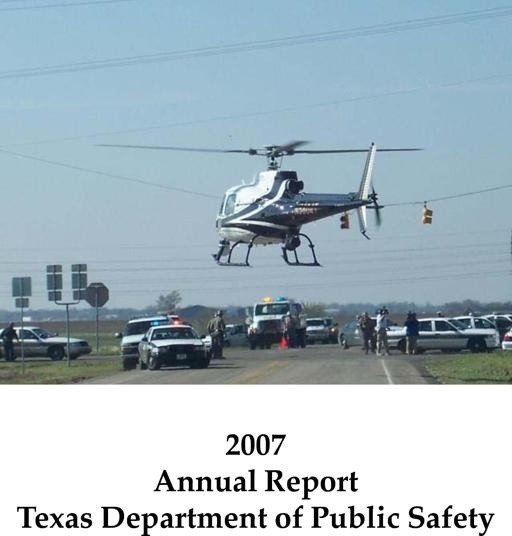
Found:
[338,314,403,351]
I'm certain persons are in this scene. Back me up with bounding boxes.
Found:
[0,321,19,362]
[207,311,218,359]
[329,322,339,344]
[436,312,445,317]
[210,310,226,359]
[355,311,377,355]
[403,310,419,356]
[375,305,392,356]
[280,311,307,349]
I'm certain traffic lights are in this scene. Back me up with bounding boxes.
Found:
[340,212,350,229]
[420,208,433,225]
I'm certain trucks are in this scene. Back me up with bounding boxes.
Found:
[244,296,311,350]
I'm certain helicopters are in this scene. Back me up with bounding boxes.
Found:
[91,138,430,270]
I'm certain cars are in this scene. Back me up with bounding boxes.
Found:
[1,325,91,362]
[134,322,212,372]
[200,320,248,349]
[352,307,512,352]
[167,314,184,324]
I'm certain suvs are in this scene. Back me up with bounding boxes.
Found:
[113,314,175,370]
[325,318,340,345]
[305,316,331,343]
